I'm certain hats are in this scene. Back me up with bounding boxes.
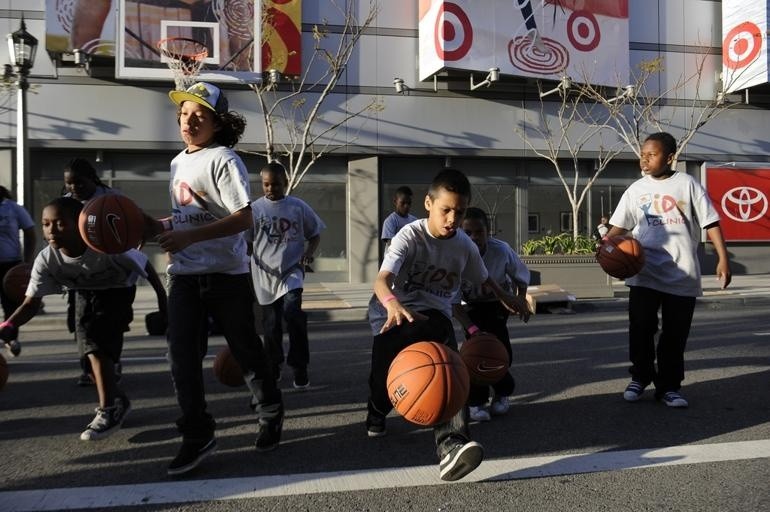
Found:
[169,81,228,115]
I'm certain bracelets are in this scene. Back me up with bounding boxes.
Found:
[160,218,171,233]
[0,322,14,327]
[602,236,609,240]
[383,294,396,305]
[467,325,479,335]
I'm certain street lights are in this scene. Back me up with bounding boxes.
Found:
[6,13,38,266]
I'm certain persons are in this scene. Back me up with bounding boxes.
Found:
[452,207,530,423]
[366,167,529,481]
[381,185,419,257]
[0,197,169,442]
[141,82,283,476]
[242,163,327,389]
[595,132,731,408]
[0,184,37,358]
[60,156,124,386]
[592,216,609,240]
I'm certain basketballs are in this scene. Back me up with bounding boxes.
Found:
[3,263,33,302]
[77,192,146,254]
[595,236,644,278]
[214,347,247,386]
[386,341,470,425]
[460,334,510,385]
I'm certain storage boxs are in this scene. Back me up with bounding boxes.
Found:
[520,282,577,314]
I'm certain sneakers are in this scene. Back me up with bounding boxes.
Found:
[624,380,645,401]
[5,341,21,357]
[77,362,310,475]
[368,397,510,481]
[661,391,688,407]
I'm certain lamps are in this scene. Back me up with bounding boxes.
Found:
[393,79,408,93]
[266,69,280,92]
[1,65,13,80]
[468,67,500,90]
[73,48,86,68]
[605,85,636,104]
[538,77,572,99]
